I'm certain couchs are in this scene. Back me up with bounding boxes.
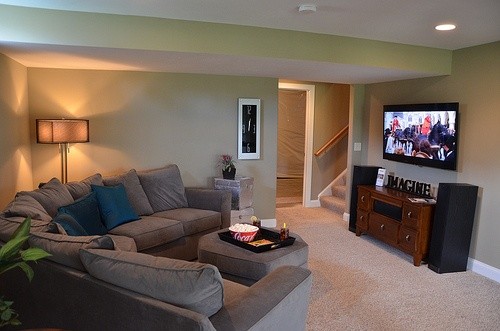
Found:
[0,186,311,331]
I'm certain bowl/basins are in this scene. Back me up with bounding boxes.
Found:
[229,225,260,243]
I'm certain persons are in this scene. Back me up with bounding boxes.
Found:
[384,116,455,162]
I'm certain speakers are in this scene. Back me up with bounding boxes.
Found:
[349,165,383,233]
[427,182,479,274]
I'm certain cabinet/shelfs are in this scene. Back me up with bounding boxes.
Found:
[355,185,437,266]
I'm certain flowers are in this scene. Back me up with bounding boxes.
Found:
[220,154,234,172]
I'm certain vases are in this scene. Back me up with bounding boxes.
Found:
[223,167,236,180]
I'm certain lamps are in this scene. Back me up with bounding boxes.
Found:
[36,119,90,184]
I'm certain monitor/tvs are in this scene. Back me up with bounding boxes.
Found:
[382,102,459,172]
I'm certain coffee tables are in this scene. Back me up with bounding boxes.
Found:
[196,226,309,287]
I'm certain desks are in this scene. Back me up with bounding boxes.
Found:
[214,175,255,211]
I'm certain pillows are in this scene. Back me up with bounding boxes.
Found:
[4,164,226,319]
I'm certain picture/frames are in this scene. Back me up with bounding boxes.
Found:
[237,97,260,160]
